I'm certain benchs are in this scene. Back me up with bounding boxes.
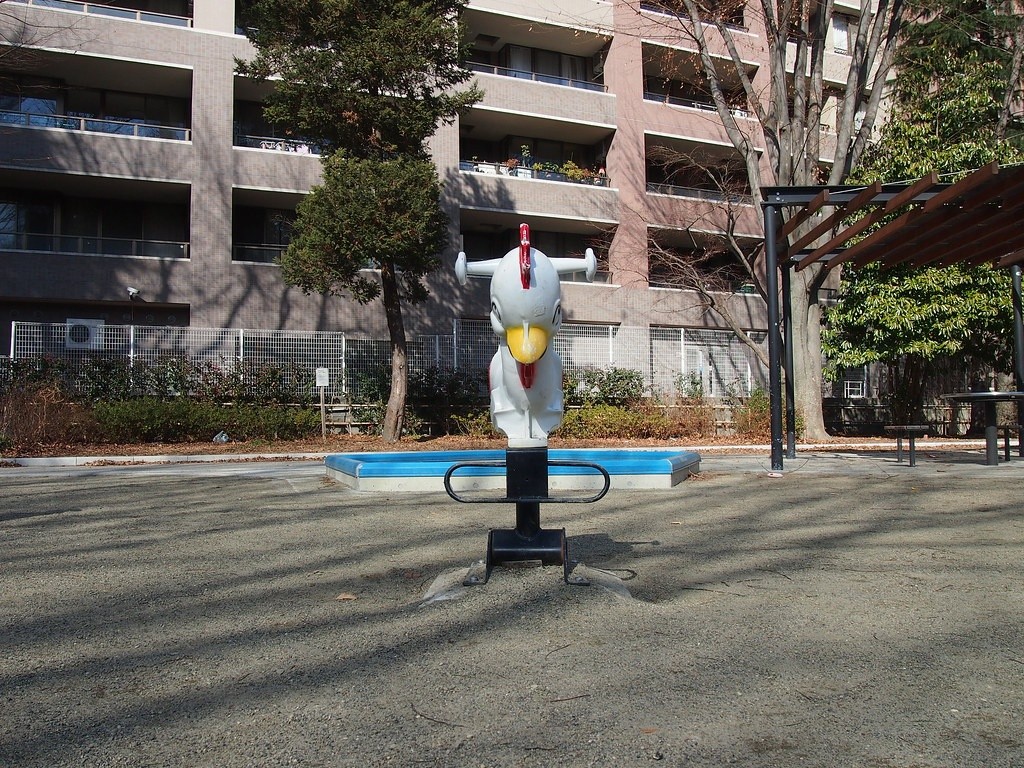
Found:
[998,424,1023,461]
[884,425,928,467]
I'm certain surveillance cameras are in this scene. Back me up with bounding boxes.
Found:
[127,287,140,294]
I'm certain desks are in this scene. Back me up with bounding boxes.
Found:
[939,391,1024,465]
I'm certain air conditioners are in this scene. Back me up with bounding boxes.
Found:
[64,317,104,351]
